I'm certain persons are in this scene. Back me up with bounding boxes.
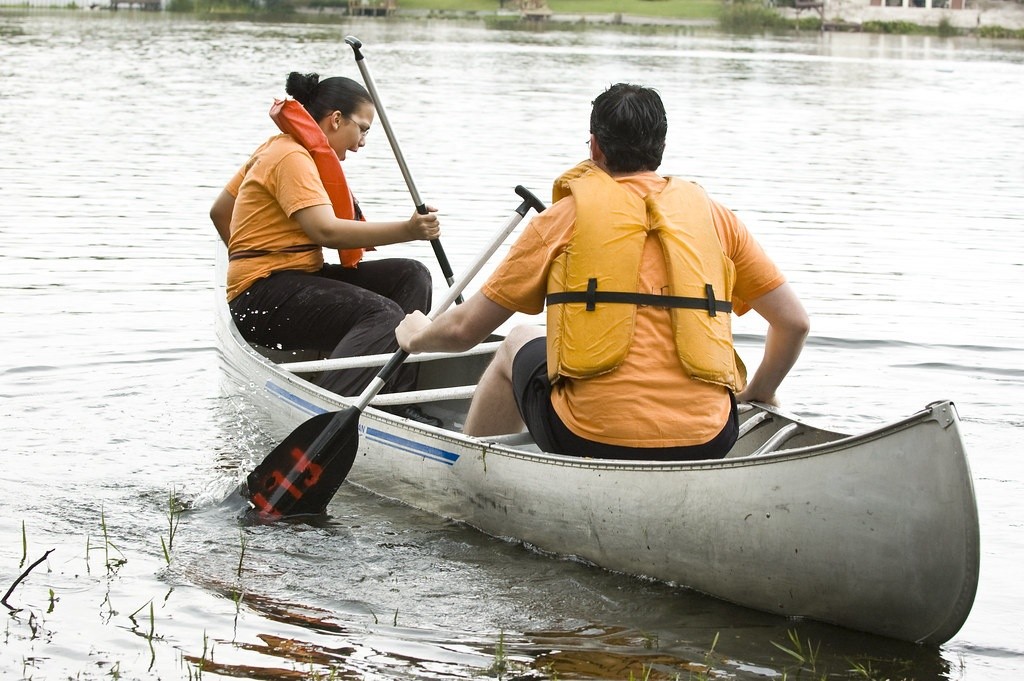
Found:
[394,82,810,460]
[210,71,445,428]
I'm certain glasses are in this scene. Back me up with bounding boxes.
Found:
[343,113,369,138]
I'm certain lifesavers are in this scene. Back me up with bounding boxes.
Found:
[270,98,377,267]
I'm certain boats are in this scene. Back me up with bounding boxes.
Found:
[212,232,984,648]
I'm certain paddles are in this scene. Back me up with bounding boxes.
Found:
[215,184,546,526]
[343,35,506,343]
